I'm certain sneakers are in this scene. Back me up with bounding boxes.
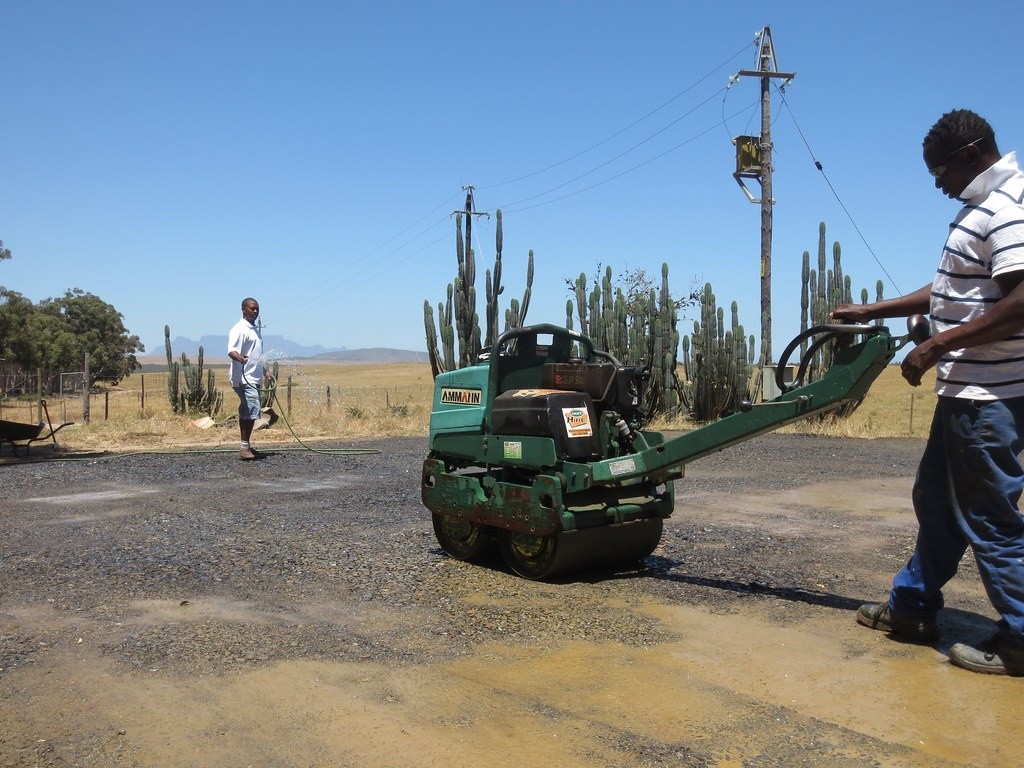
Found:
[238,447,266,461]
[855,600,938,637]
[948,632,1024,674]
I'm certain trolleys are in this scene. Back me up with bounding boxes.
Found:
[0,420,75,456]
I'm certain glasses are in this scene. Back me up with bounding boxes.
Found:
[928,136,985,180]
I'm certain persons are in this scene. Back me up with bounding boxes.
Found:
[228,298,274,459]
[829,108,1024,676]
